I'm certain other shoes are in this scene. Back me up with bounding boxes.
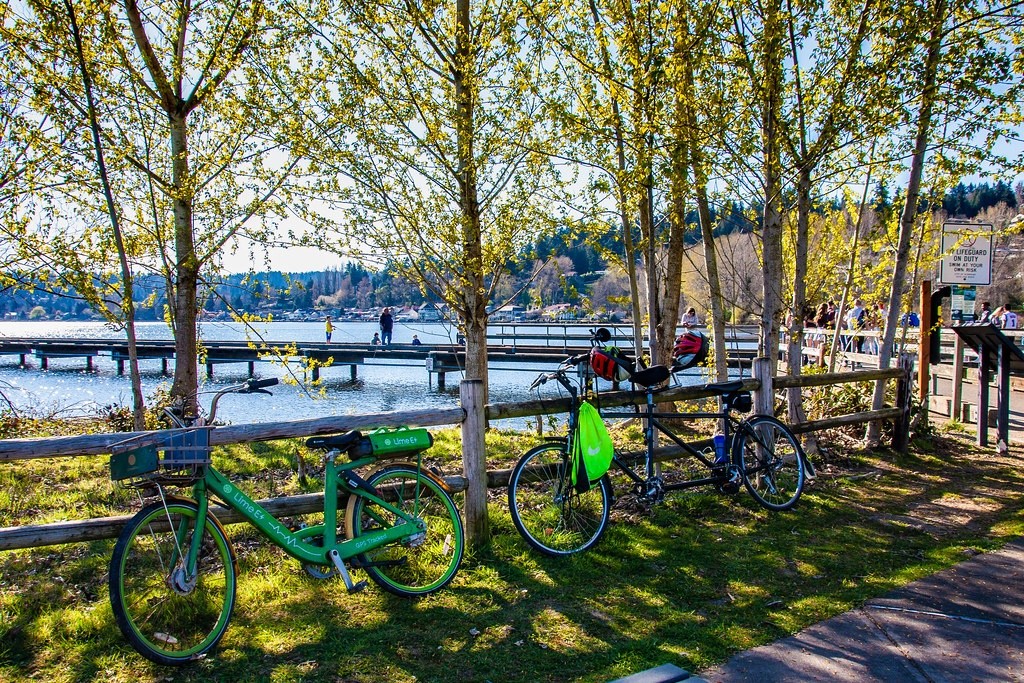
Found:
[842,363,848,367]
[856,364,862,367]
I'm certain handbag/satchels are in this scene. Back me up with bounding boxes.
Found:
[571,401,614,494]
[857,310,866,328]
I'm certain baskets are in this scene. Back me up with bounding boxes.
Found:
[105,426,217,490]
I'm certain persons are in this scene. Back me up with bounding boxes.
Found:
[979,302,1017,341]
[784,298,919,368]
[379,307,393,351]
[325,316,333,344]
[412,335,421,345]
[372,333,381,345]
[682,308,697,332]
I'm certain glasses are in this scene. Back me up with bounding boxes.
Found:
[689,312,694,315]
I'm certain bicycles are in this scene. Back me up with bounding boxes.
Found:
[505,328,818,559]
[106,377,468,668]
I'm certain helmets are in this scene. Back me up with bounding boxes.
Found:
[671,331,710,371]
[589,346,635,383]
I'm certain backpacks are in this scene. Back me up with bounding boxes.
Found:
[909,312,919,327]
